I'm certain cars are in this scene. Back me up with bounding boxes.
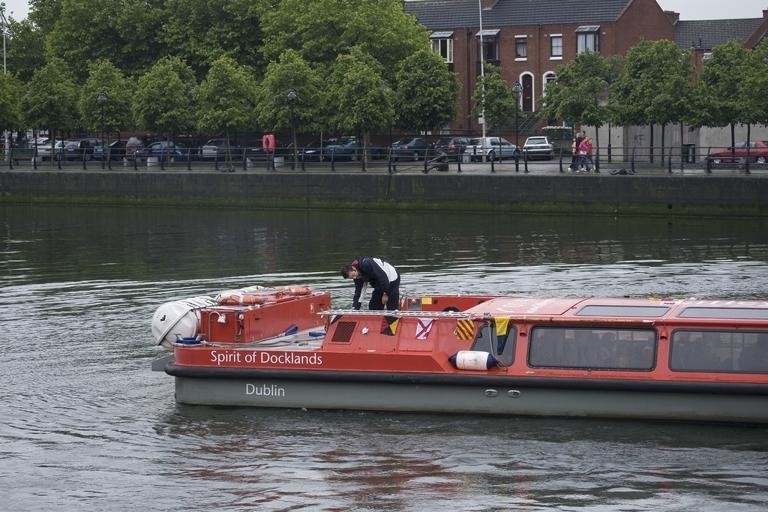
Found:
[135,141,190,164]
[26,137,48,149]
[36,138,69,160]
[465,137,522,162]
[198,138,240,160]
[325,135,386,160]
[93,140,128,162]
[1,134,24,153]
[524,136,556,160]
[297,138,327,161]
[705,140,768,165]
[245,140,285,162]
[387,138,433,160]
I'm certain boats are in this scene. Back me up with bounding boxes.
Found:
[148,284,768,424]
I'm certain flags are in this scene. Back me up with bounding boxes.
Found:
[379,314,400,336]
[494,315,510,338]
[454,317,475,341]
[413,317,436,341]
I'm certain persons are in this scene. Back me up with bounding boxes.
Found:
[426,146,450,173]
[585,138,596,170]
[579,137,592,172]
[341,255,401,311]
[567,132,583,170]
[485,145,497,162]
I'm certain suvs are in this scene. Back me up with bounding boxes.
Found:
[125,137,164,161]
[63,138,102,161]
[434,137,472,162]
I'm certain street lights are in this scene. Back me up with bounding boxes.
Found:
[95,93,109,168]
[0,3,13,75]
[288,91,298,170]
[511,81,523,171]
